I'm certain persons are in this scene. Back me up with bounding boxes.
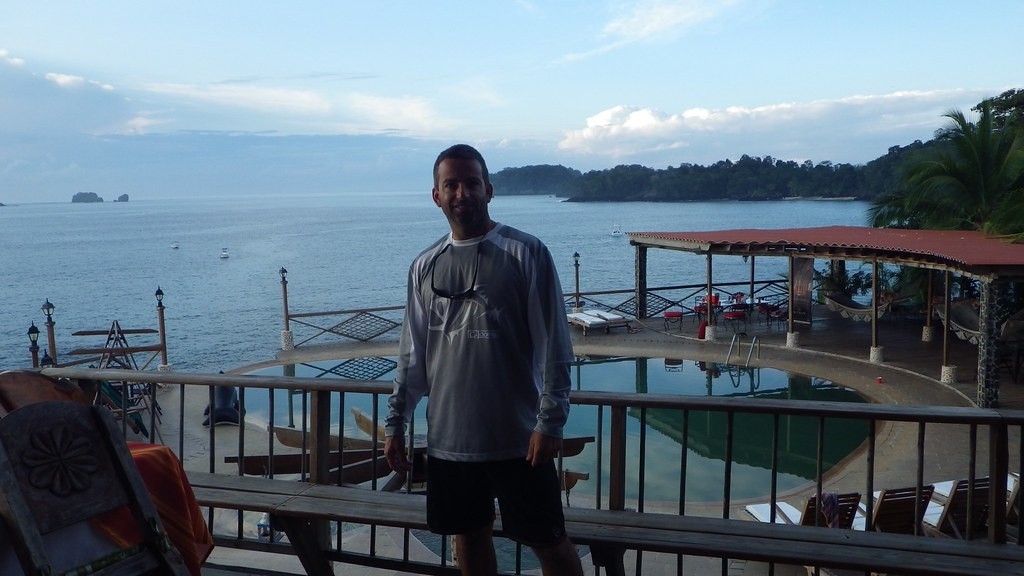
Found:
[382,145,584,576]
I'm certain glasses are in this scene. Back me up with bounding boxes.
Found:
[431,241,482,299]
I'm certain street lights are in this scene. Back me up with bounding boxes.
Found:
[154,284,168,365]
[278,266,291,331]
[26,319,41,368]
[571,251,581,308]
[40,297,57,369]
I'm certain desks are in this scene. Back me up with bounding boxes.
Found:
[699,300,754,308]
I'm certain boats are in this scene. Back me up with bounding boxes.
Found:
[611,224,622,237]
[220,247,229,258]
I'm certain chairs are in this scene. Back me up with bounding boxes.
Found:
[739,470,1024,576]
[665,358,684,373]
[582,310,634,335]
[566,313,607,336]
[693,292,751,334]
[663,301,684,332]
[757,295,789,329]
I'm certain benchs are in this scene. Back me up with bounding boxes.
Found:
[181,469,335,576]
[273,477,1024,576]
